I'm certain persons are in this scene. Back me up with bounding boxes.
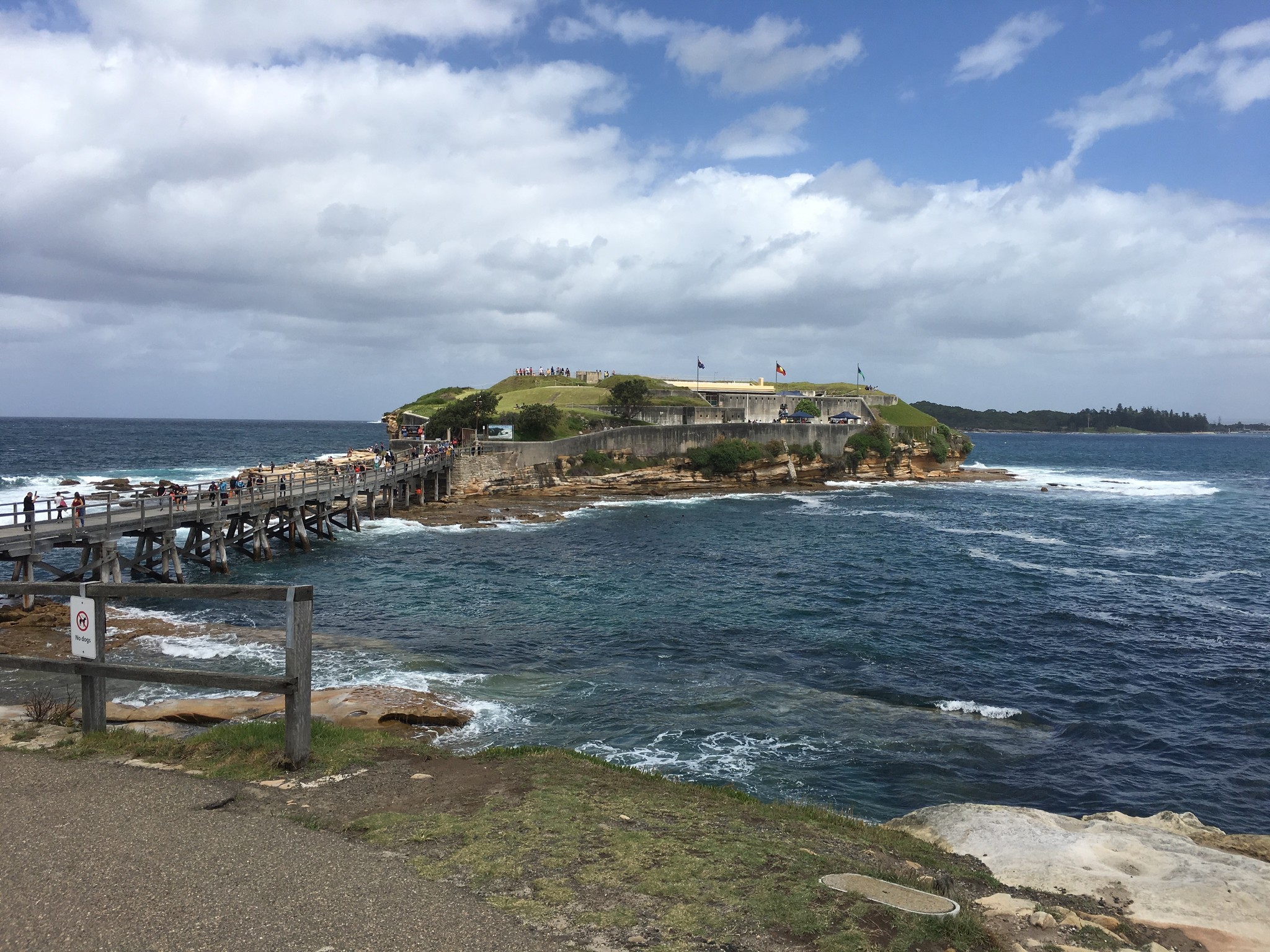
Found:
[327,437,484,486]
[604,424,623,431]
[156,483,188,511]
[515,366,570,377]
[208,475,264,507]
[865,385,878,391]
[393,484,426,503]
[727,404,846,424]
[291,471,295,486]
[514,403,525,408]
[54,491,85,531]
[288,462,297,467]
[23,492,38,531]
[596,370,615,379]
[271,461,274,473]
[305,457,308,467]
[258,460,262,472]
[863,419,871,425]
[279,474,286,497]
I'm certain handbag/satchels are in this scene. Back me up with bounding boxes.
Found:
[169,492,175,501]
[338,467,342,477]
[237,481,242,489]
[60,496,67,507]
[223,493,228,504]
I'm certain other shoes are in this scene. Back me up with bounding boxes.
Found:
[176,510,180,511]
[29,528,36,531]
[76,525,79,528]
[61,521,64,523]
[210,495,285,507]
[333,479,364,486]
[182,510,186,512]
[160,508,163,511]
[56,522,59,524]
[23,528,30,532]
[82,525,84,527]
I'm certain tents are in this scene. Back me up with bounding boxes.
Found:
[787,410,814,420]
[830,411,861,424]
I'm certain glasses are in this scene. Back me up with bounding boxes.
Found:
[283,475,285,476]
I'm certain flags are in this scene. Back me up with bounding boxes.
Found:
[698,358,705,369]
[858,367,865,381]
[777,363,786,376]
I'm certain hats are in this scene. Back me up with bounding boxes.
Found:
[361,461,363,463]
[219,479,223,482]
[211,481,215,484]
[349,464,351,465]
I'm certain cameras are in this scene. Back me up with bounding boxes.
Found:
[35,491,40,498]
